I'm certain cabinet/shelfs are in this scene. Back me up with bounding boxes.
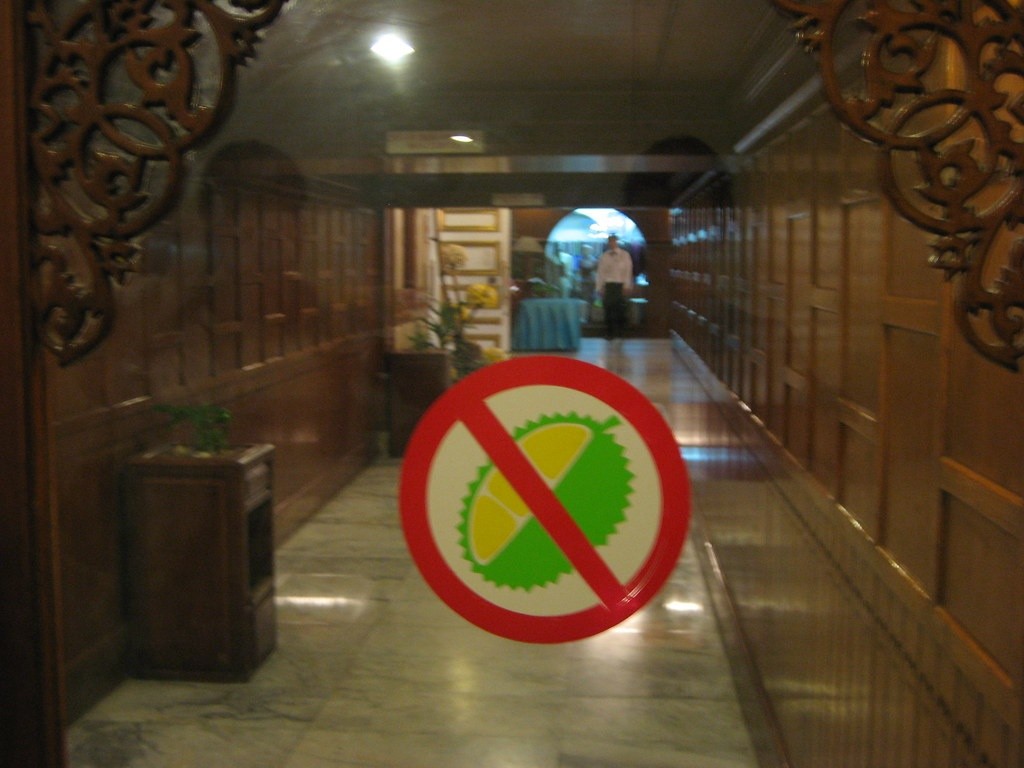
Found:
[123,441,276,684]
[386,347,448,455]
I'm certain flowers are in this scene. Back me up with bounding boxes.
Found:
[406,239,507,382]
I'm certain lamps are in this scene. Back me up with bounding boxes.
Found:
[512,235,543,298]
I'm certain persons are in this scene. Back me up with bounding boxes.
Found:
[579,245,598,304]
[553,242,575,297]
[596,235,633,339]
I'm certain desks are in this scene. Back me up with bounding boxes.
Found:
[509,297,587,350]
